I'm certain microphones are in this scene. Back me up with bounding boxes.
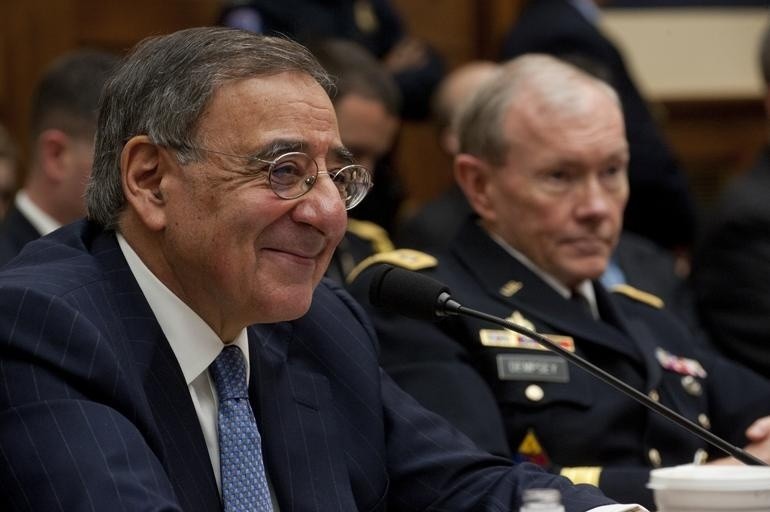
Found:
[368,264,770,465]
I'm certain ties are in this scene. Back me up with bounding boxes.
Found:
[207,341,275,511]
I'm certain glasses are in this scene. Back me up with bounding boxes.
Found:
[171,143,374,211]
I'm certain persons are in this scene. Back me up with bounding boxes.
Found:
[345,52,770,508]
[0,44,119,272]
[0,27,617,512]
[687,26,770,377]
[210,0,703,297]
